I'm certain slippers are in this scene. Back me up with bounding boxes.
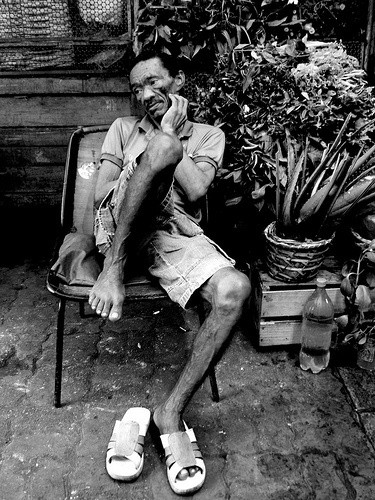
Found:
[159,420,206,495]
[104,407,151,481]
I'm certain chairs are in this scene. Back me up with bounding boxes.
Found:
[46,121,222,409]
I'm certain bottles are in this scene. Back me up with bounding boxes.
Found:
[298,278,334,374]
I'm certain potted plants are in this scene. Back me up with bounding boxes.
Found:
[262,110,375,282]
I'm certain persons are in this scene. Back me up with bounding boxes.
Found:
[80,48,255,494]
